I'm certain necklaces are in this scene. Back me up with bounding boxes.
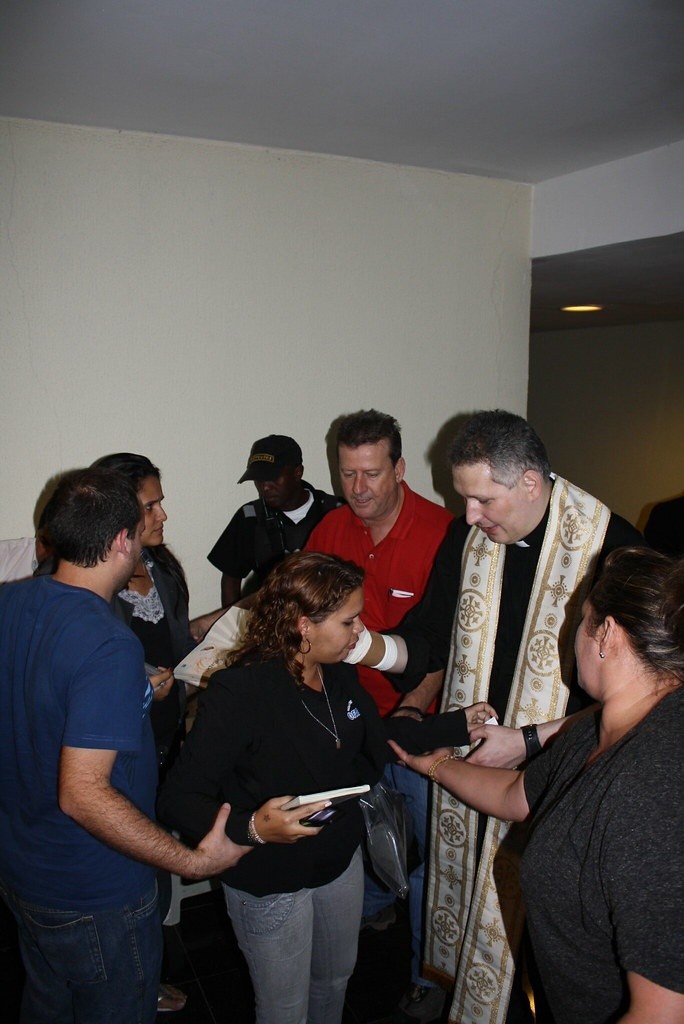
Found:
[299,665,341,749]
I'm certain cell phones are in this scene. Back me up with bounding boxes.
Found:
[300,807,339,827]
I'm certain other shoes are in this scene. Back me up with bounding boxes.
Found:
[156,984,186,1012]
[395,981,448,1024]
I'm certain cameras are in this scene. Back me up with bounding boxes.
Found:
[144,663,166,688]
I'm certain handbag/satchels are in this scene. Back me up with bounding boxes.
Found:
[363,784,424,899]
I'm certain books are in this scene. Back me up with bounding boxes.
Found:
[281,785,370,826]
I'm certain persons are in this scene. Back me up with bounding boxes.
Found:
[208,434,348,607]
[0,467,254,1024]
[158,551,498,1024]
[385,549,684,1024]
[92,452,263,1024]
[301,410,459,1024]
[342,410,641,1024]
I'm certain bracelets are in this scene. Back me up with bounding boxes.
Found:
[522,725,542,759]
[400,706,423,717]
[429,755,452,780]
[370,635,398,672]
[246,810,265,847]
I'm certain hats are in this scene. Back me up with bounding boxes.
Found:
[237,434,302,484]
